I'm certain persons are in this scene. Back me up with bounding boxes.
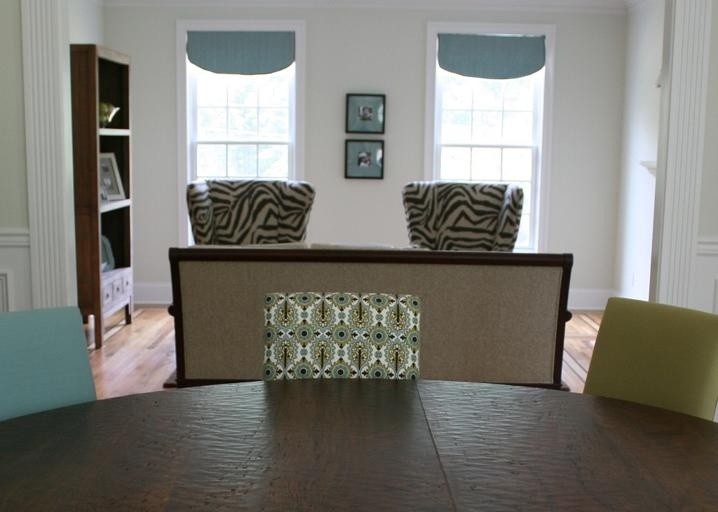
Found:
[361,106,373,121]
[358,153,371,167]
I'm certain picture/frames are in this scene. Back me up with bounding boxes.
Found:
[344,92,385,179]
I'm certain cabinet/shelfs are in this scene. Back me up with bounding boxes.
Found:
[68,43,133,350]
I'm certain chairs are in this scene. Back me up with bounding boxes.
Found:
[1,305,100,421]
[580,294,716,423]
[186,178,317,244]
[262,288,424,384]
[401,179,527,253]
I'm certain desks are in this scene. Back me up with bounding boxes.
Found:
[166,243,576,393]
[4,379,716,511]
